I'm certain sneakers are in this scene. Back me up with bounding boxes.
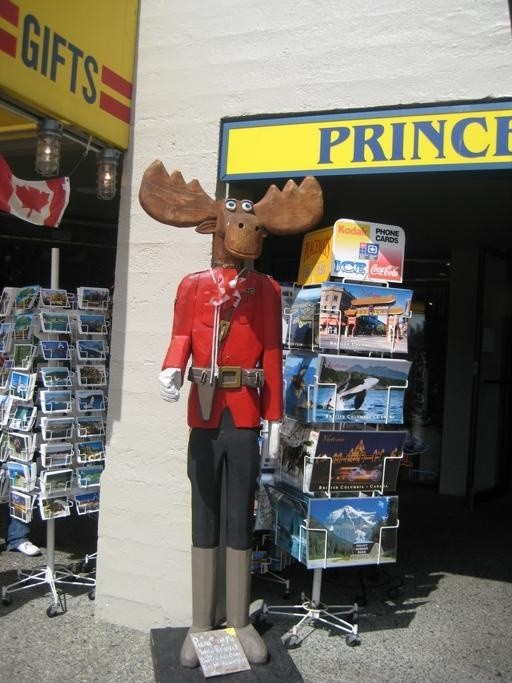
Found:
[17,541,41,556]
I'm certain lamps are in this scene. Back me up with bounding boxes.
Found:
[95,147,122,202]
[34,117,65,178]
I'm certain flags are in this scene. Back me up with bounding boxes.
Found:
[0,152,72,229]
